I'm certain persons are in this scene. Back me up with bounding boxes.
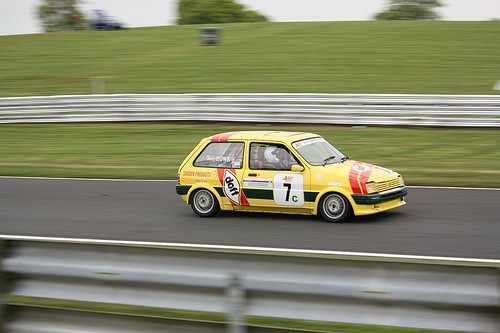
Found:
[263,146,295,170]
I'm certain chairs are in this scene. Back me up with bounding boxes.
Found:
[256,145,267,169]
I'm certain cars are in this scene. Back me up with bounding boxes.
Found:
[174,130,409,224]
